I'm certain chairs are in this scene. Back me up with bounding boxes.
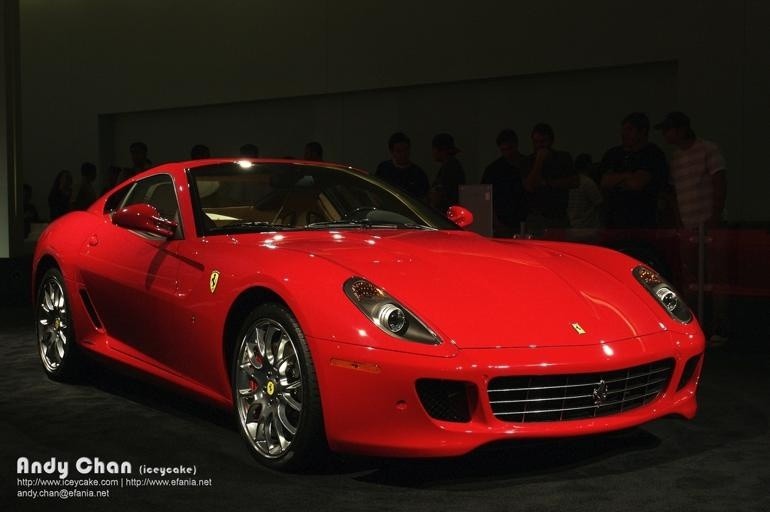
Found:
[146,181,190,219]
[490,222,769,355]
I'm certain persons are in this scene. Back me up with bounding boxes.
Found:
[22,183,39,239]
[46,111,732,349]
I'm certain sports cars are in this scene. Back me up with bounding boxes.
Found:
[30,156,706,470]
